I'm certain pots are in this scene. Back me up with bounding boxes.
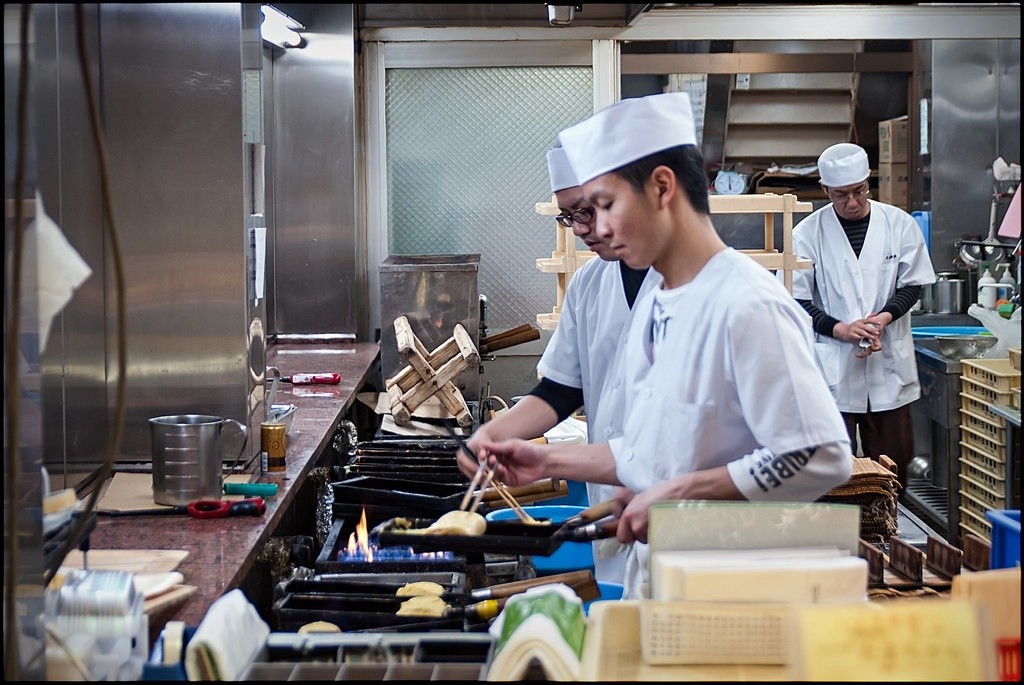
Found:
[271,435,620,633]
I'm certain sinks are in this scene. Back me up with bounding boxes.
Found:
[914,338,990,428]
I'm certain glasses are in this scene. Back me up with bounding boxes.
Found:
[828,184,869,203]
[555,205,595,228]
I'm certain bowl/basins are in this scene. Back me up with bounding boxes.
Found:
[912,326,990,339]
[486,505,594,570]
[582,584,624,616]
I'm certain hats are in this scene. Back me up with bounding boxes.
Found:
[817,142,871,188]
[556,90,698,187]
[546,145,580,193]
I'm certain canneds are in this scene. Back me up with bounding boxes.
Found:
[260,421,287,475]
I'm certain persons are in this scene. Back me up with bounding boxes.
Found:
[774,143,937,508]
[480,91,853,602]
[457,146,665,587]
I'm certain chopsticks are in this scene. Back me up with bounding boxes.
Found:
[442,422,535,524]
[461,449,501,513]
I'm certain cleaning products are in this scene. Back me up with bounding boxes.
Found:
[976,263,998,312]
[994,262,1017,304]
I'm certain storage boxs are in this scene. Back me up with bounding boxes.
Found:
[636,581,793,664]
[877,112,908,211]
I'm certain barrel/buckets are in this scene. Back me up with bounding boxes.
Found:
[936,279,966,314]
[938,271,959,280]
[919,284,937,313]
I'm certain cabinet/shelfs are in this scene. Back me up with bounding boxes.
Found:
[534,192,819,332]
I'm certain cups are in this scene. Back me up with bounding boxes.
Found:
[149,414,248,506]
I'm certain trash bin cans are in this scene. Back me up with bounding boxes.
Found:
[984,509,1021,569]
